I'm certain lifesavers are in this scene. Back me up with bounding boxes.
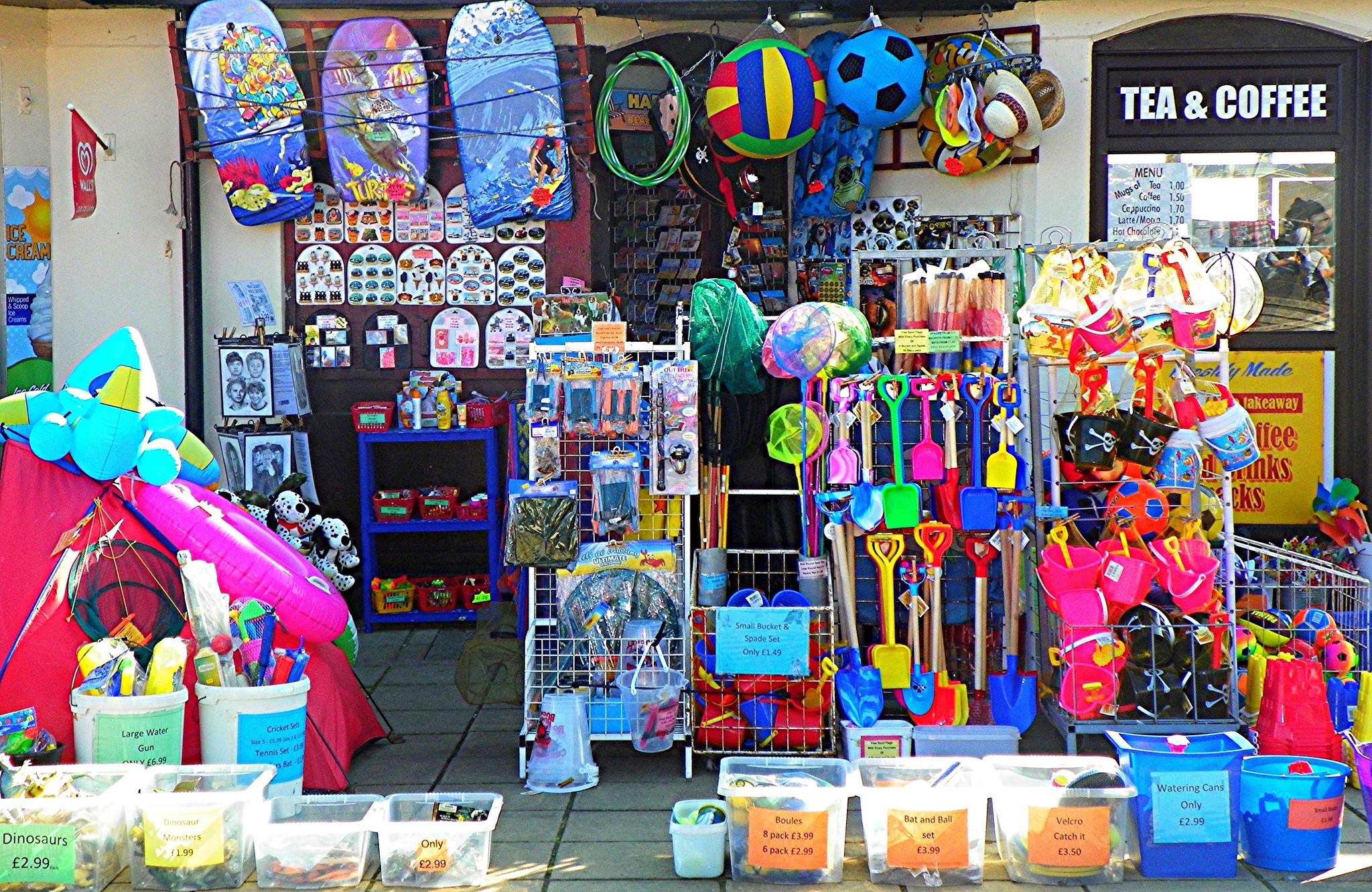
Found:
[898,32,1015,178]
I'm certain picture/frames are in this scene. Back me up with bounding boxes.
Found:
[217,346,274,418]
[240,434,292,495]
[217,432,246,495]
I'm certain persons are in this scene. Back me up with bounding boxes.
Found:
[309,338,316,345]
[340,356,350,366]
[251,445,282,494]
[225,443,243,491]
[226,379,248,411]
[247,382,268,411]
[245,352,266,383]
[225,351,246,382]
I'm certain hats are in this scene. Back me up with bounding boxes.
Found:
[934,66,1073,150]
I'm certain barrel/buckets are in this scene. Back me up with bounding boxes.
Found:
[68,637,189,768]
[1053,382,1263,495]
[524,616,682,795]
[1344,730,1372,840]
[195,675,310,820]
[1237,755,1352,871]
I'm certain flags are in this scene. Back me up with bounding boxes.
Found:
[63,102,114,222]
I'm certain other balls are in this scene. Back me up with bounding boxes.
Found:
[1039,443,1344,720]
[827,29,927,132]
[705,40,830,160]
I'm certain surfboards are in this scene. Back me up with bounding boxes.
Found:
[185,0,317,227]
[444,0,575,228]
[319,16,431,205]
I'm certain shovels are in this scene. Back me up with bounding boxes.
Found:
[691,244,1235,737]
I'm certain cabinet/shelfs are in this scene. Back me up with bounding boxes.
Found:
[357,425,511,631]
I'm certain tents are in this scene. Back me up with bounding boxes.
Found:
[0,426,402,793]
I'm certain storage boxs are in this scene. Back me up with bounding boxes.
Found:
[984,754,1137,887]
[839,719,913,760]
[849,758,990,886]
[670,799,728,880]
[717,756,856,885]
[375,793,503,890]
[123,765,275,892]
[1107,727,1255,881]
[253,793,381,890]
[2,763,145,892]
[912,725,1022,798]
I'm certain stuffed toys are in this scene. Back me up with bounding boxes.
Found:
[220,469,358,592]
